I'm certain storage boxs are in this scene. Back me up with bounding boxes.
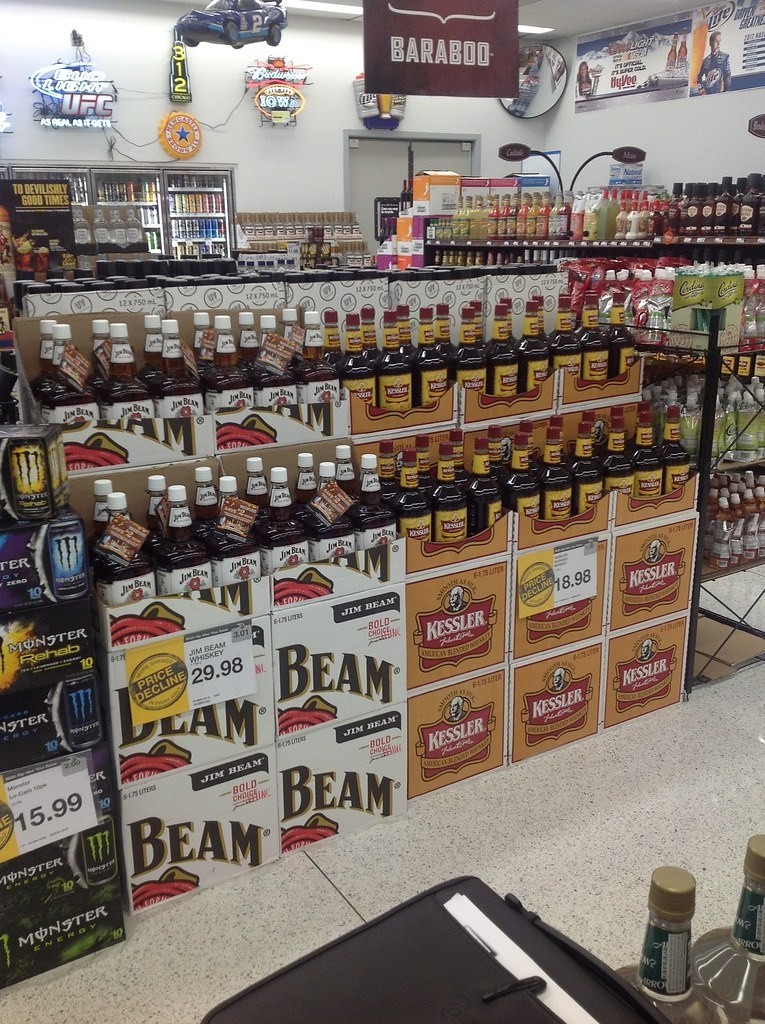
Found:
[508,533,612,665]
[109,743,281,918]
[274,702,407,859]
[460,176,490,210]
[64,459,270,654]
[504,172,550,207]
[1,424,71,531]
[669,276,743,356]
[606,510,700,635]
[221,436,408,614]
[405,553,512,695]
[490,178,520,208]
[9,274,645,475]
[92,612,277,791]
[0,817,127,989]
[376,206,453,270]
[508,639,604,766]
[0,667,105,772]
[0,504,90,614]
[0,740,115,822]
[512,488,612,556]
[269,581,407,742]
[611,468,700,531]
[413,169,460,215]
[407,665,510,804]
[0,591,95,696]
[398,506,513,580]
[601,610,691,734]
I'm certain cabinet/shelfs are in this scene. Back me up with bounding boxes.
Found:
[425,233,765,266]
[0,159,237,281]
[596,321,765,703]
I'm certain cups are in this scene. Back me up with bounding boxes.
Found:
[689,6,710,94]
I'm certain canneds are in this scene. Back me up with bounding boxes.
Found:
[12,437,118,887]
[97,174,226,259]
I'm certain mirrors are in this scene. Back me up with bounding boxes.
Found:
[499,42,569,120]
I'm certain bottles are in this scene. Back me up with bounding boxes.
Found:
[611,830,765,1024]
[35,288,764,607]
[238,211,372,273]
[433,173,765,355]
[665,28,689,73]
